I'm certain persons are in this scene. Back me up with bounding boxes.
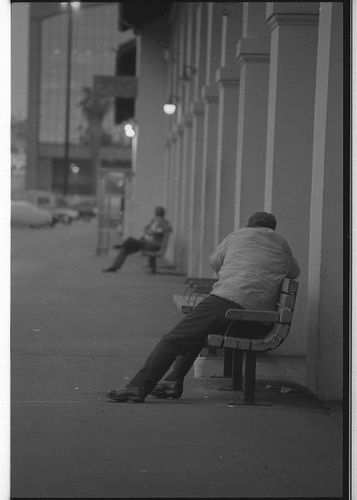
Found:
[103,206,170,273]
[105,212,300,405]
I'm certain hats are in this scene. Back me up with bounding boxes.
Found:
[248,212,276,230]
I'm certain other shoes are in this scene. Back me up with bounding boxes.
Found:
[149,379,183,398]
[104,266,115,272]
[114,244,121,249]
[106,387,145,402]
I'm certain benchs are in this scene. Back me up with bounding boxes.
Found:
[142,225,170,274]
[173,277,299,407]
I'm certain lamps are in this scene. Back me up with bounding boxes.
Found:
[163,94,179,115]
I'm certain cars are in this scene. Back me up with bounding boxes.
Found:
[10,191,99,229]
